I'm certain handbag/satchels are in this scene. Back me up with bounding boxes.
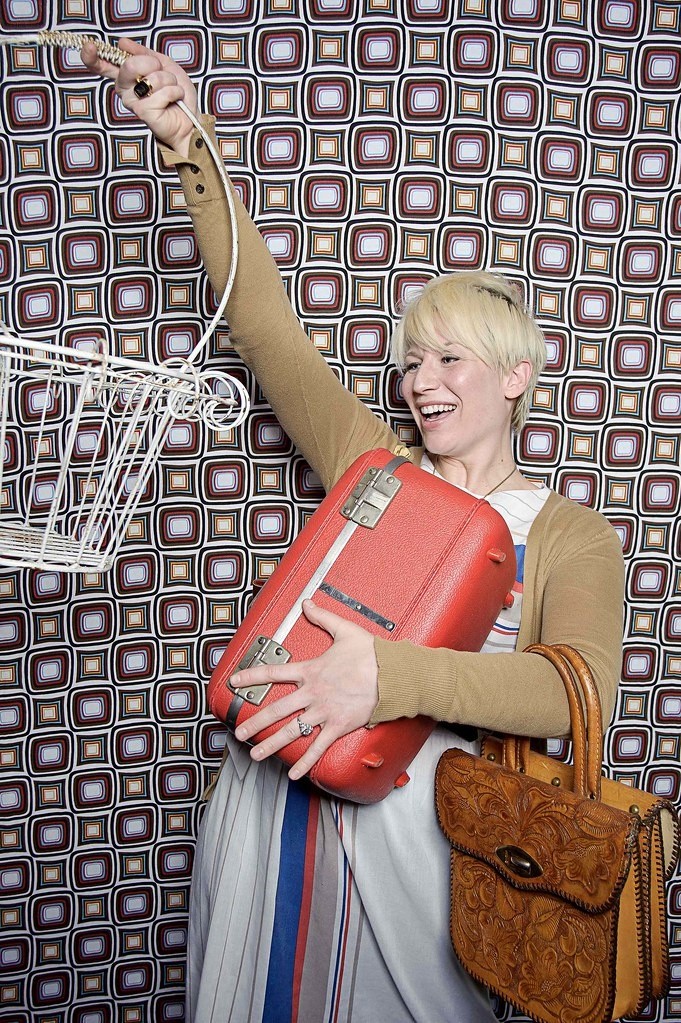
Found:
[433,644,681,1023]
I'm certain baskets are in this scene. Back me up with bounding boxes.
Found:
[0,35,250,571]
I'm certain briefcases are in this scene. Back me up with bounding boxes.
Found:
[206,448,517,806]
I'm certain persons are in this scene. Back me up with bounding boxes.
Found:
[79,36,628,1023]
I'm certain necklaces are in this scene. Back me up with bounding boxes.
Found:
[424,459,518,500]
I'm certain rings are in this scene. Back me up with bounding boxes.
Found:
[296,714,314,737]
[133,78,153,99]
